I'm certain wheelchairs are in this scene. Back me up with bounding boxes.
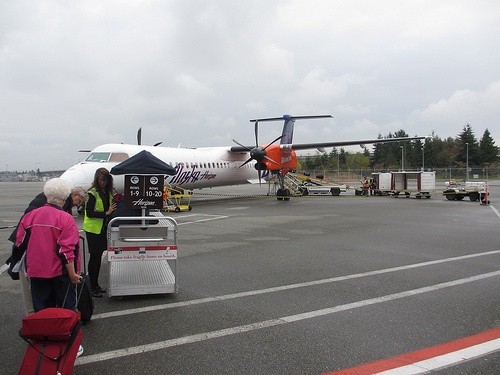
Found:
[42,128,269,213]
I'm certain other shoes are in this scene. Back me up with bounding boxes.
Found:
[91,289,102,297]
[95,286,106,293]
[77,345,84,357]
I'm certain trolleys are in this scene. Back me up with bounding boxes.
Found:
[97,216,179,296]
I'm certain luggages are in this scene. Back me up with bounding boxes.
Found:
[18,277,84,375]
[66,236,94,321]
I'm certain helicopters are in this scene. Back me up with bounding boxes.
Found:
[230,114,434,200]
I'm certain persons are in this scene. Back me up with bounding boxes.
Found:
[361,177,373,196]
[84,168,117,298]
[8,185,89,313]
[8,178,84,359]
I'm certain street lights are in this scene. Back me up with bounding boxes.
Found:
[421,148,424,171]
[400,146,404,172]
[466,143,469,172]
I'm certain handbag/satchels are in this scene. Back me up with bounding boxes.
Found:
[20,308,82,361]
